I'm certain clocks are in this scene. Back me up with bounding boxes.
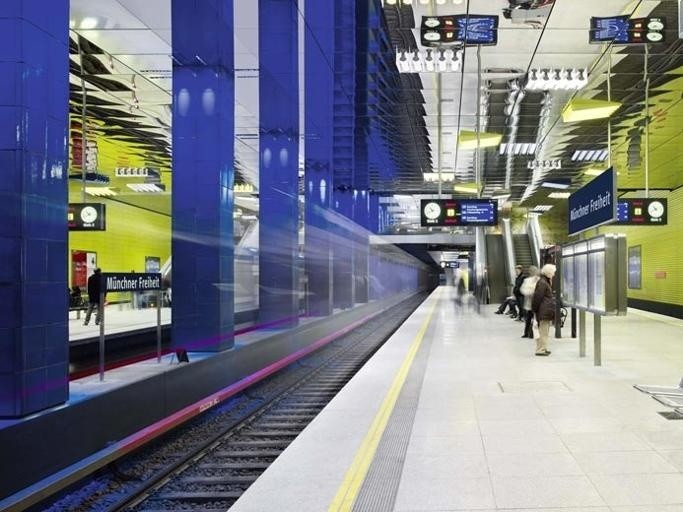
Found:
[648,202,663,218]
[80,207,97,223]
[424,202,440,219]
[647,22,663,41]
[424,19,441,42]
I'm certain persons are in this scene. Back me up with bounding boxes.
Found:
[493,263,557,356]
[68,267,107,325]
[159,276,172,307]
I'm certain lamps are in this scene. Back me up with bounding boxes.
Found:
[398,47,460,72]
[531,69,584,90]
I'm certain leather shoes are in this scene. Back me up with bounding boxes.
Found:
[494,310,502,314]
[546,351,551,354]
[522,335,527,338]
[536,353,547,356]
